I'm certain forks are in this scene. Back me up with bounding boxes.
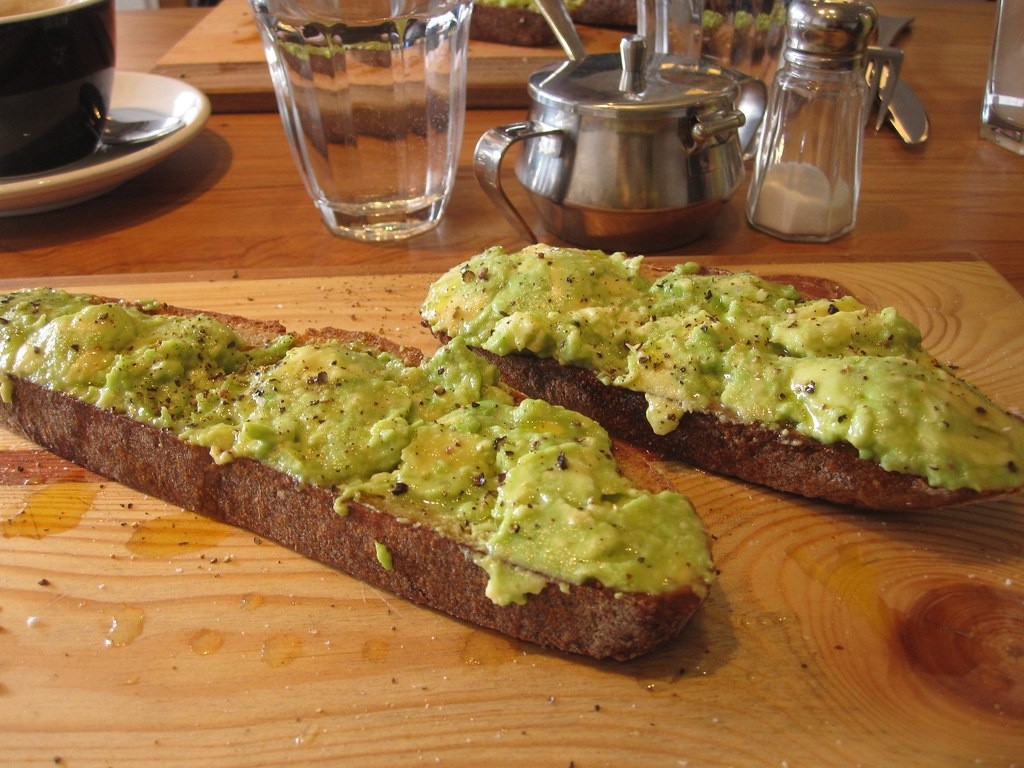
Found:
[862,15,916,131]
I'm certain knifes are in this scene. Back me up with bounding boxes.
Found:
[864,58,931,148]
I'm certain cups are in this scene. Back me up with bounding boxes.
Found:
[978,0,1024,155]
[473,50,768,252]
[251,0,474,242]
[637,1,705,59]
[746,0,881,242]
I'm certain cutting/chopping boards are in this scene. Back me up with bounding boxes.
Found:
[146,0,636,112]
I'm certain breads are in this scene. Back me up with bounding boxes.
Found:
[0,293,718,663]
[462,0,766,46]
[423,322,1024,510]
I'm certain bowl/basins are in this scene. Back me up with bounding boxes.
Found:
[1,1,118,180]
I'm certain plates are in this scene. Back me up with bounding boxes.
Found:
[0,69,211,217]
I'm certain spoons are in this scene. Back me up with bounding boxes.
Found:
[94,107,184,149]
